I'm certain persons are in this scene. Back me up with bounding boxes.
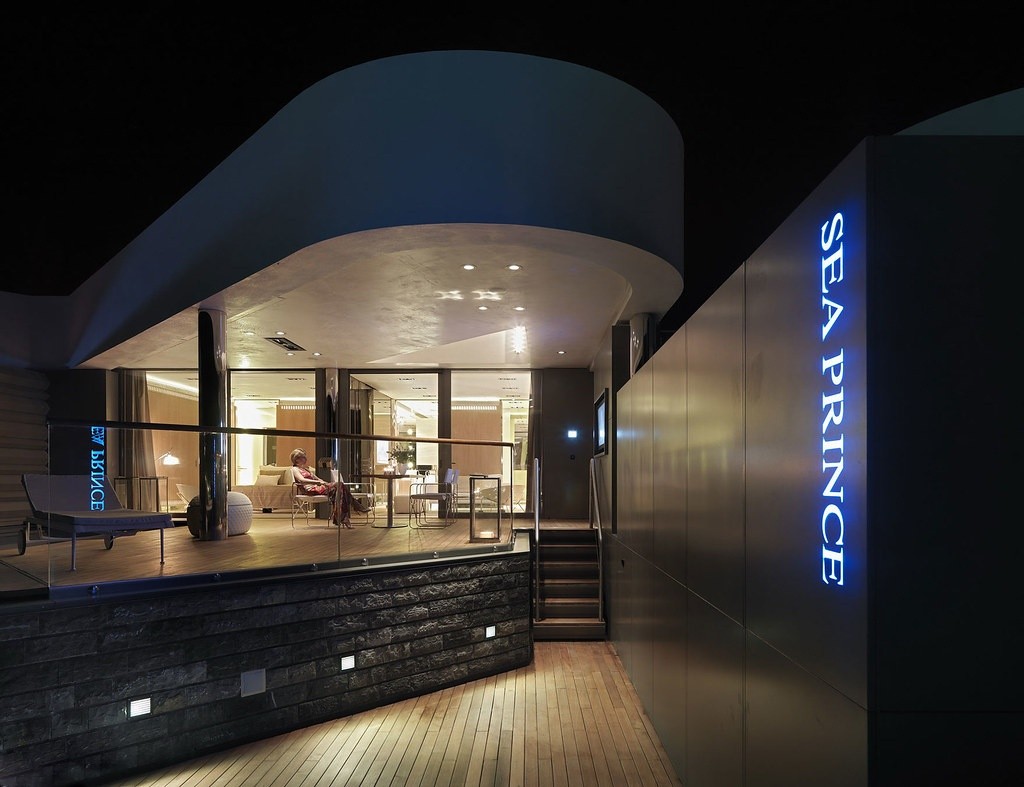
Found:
[290,447,372,529]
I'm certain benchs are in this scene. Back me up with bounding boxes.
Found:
[18,474,174,568]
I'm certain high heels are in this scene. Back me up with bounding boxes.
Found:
[354,508,371,516]
[343,522,355,529]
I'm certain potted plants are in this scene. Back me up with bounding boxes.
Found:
[386,443,413,475]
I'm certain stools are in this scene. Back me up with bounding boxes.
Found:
[394,495,423,514]
[186,492,253,536]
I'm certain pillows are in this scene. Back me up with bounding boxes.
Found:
[255,474,281,486]
[260,469,287,484]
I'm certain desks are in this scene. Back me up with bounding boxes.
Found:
[349,474,410,528]
[112,477,169,511]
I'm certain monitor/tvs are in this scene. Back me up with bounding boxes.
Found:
[596,398,605,451]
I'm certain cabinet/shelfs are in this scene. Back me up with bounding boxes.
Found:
[468,477,501,543]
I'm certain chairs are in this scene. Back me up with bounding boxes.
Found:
[330,469,376,525]
[177,484,199,507]
[409,469,452,529]
[418,469,459,526]
[479,485,526,512]
[290,469,344,528]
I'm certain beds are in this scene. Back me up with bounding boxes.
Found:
[231,466,315,513]
